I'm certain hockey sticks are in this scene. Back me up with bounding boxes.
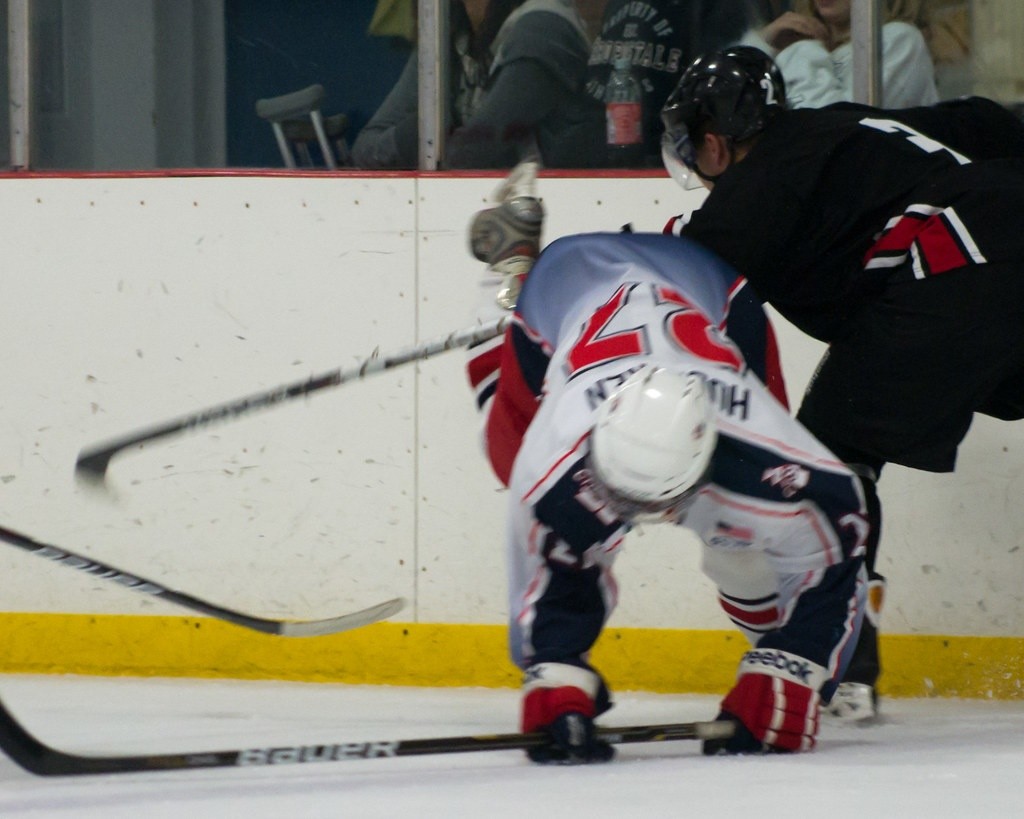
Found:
[76,319,511,485]
[1,703,736,778]
[0,527,405,640]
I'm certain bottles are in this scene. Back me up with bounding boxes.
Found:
[605,59,644,169]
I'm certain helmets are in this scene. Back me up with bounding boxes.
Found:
[660,45,786,192]
[587,366,719,528]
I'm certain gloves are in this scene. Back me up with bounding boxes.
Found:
[520,656,615,764]
[703,632,830,753]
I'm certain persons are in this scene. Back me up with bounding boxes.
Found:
[351,0,973,170]
[469,198,880,767]
[621,44,1024,726]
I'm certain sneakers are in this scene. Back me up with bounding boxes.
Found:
[471,197,544,263]
[817,681,877,721]
